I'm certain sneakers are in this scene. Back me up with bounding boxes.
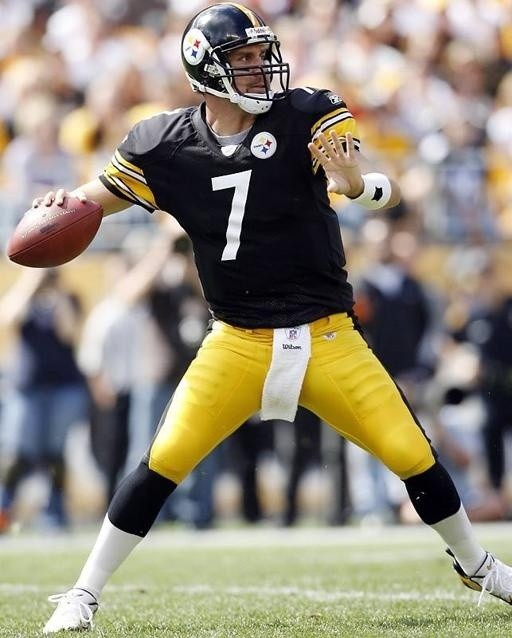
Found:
[445,546,512,603]
[42,587,100,634]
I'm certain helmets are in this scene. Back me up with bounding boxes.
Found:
[180,1,289,115]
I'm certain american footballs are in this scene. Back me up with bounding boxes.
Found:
[8,197,103,268]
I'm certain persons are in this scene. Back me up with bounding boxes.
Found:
[1,0,512,535]
[30,2,512,635]
